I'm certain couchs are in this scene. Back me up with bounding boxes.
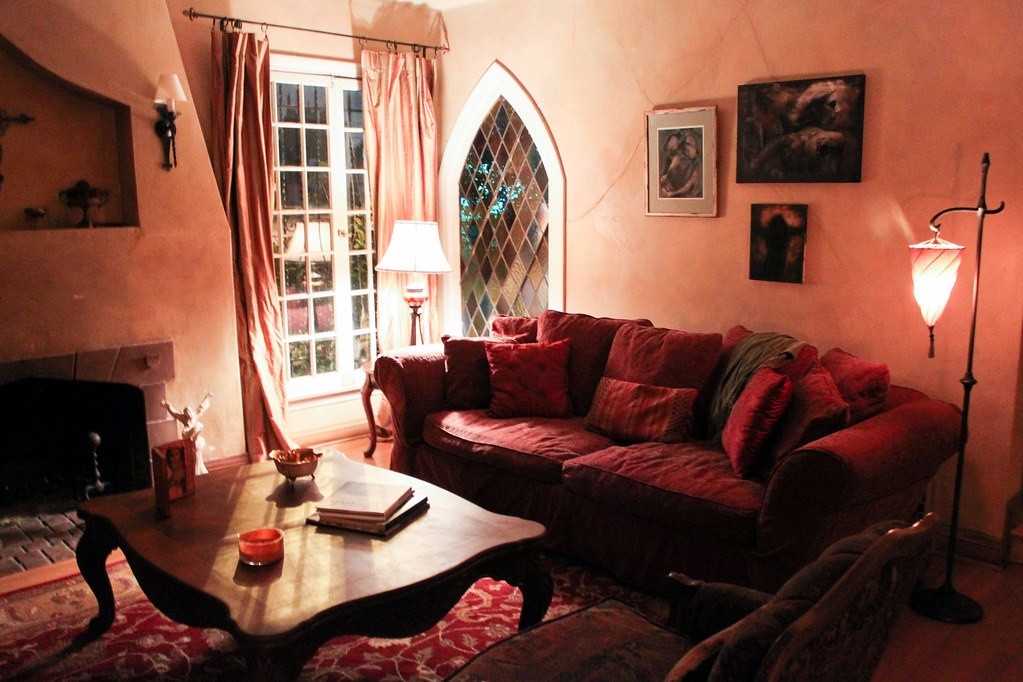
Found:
[359,338,963,591]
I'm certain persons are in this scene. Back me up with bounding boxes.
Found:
[166,449,185,499]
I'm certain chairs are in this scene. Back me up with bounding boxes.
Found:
[448,512,937,682]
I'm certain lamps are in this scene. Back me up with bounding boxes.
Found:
[152,72,188,172]
[284,223,333,329]
[375,221,453,344]
[909,154,1006,626]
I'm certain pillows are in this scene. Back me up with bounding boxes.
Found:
[443,310,890,479]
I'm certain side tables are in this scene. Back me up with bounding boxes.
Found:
[360,356,381,457]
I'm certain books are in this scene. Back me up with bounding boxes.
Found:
[306,481,429,539]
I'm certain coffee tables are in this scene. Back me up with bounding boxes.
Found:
[75,447,553,682]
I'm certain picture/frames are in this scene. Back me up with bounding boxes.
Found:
[644,105,718,219]
